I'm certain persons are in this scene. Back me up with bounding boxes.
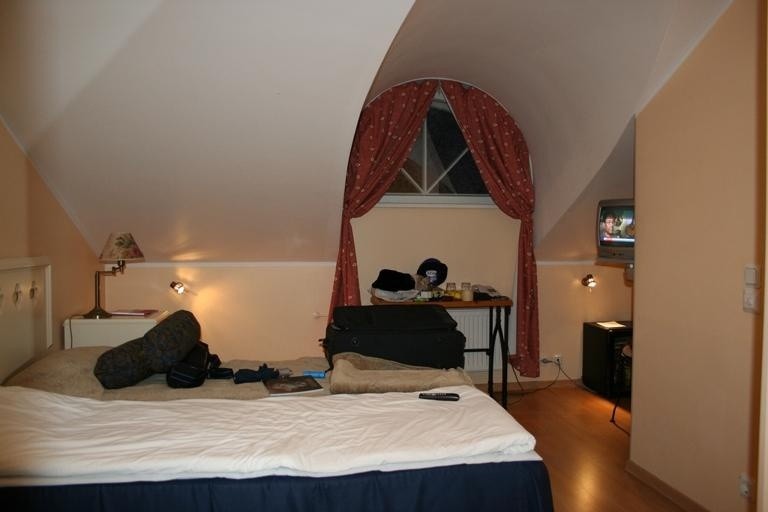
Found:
[600,212,619,241]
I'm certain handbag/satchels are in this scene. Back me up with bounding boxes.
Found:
[167,341,234,388]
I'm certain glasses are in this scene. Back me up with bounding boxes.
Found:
[604,220,615,225]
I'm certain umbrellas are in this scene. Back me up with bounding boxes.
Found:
[234,364,291,385]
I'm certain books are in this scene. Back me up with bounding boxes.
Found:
[262,376,324,396]
[110,309,158,317]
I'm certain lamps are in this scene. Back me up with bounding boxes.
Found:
[582,274,597,287]
[83,232,145,319]
[169,281,184,294]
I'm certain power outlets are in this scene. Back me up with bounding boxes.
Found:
[540,355,551,366]
[552,353,564,366]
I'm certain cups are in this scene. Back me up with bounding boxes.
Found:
[463,286,473,302]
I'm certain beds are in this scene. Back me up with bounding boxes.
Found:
[0,346,552,512]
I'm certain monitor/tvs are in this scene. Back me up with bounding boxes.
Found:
[596,199,635,259]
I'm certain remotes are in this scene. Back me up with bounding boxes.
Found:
[419,392,459,400]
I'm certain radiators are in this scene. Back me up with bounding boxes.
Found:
[446,308,505,372]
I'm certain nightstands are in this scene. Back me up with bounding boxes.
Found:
[63,308,169,349]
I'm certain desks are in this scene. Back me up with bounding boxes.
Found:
[369,294,513,409]
[581,321,632,400]
